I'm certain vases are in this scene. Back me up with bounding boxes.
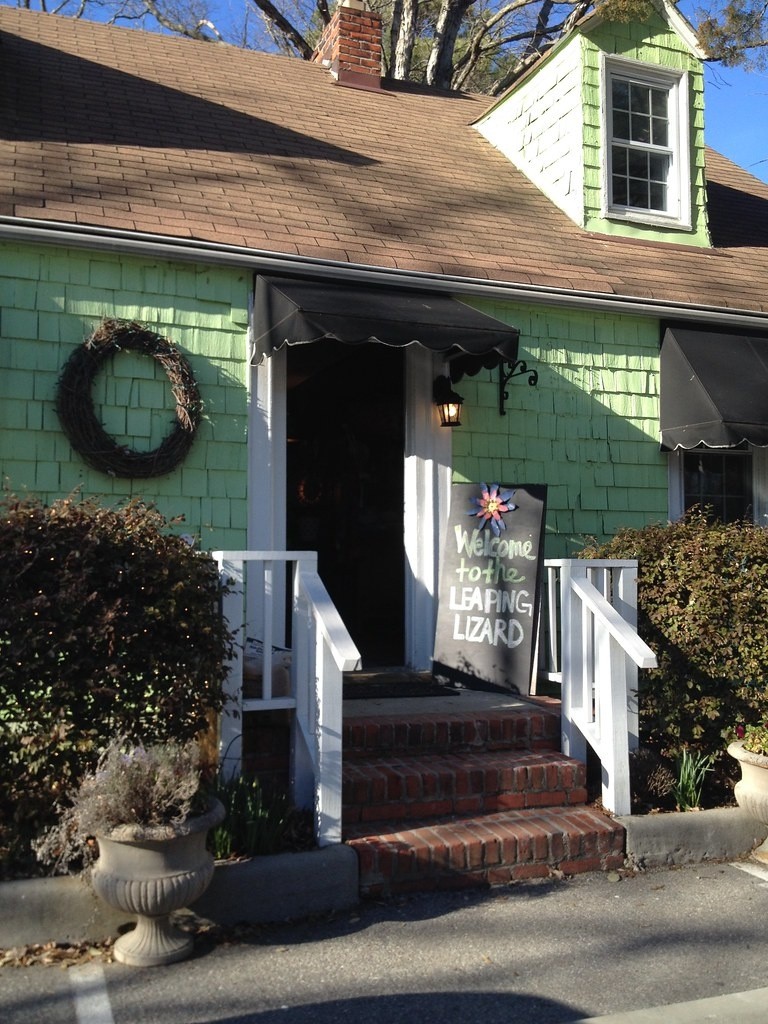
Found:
[728,741,768,864]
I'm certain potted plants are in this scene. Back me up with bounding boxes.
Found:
[30,734,225,968]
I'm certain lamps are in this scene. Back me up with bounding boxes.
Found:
[433,374,466,427]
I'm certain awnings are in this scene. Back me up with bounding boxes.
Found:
[252,277,520,384]
[659,328,768,452]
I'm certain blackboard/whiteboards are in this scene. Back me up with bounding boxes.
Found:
[432,482,550,696]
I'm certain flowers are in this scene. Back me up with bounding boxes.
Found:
[737,715,768,754]
